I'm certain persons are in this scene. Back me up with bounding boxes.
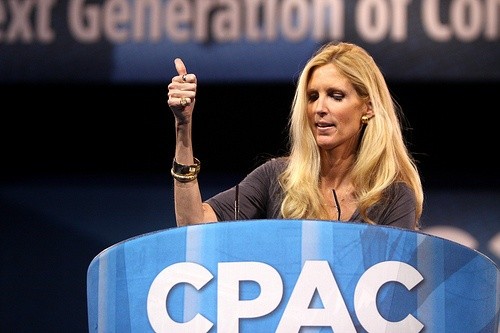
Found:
[168,41,423,231]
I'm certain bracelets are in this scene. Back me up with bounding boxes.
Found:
[171,157,201,183]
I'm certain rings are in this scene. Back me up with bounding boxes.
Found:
[181,74,188,82]
[180,96,188,107]
